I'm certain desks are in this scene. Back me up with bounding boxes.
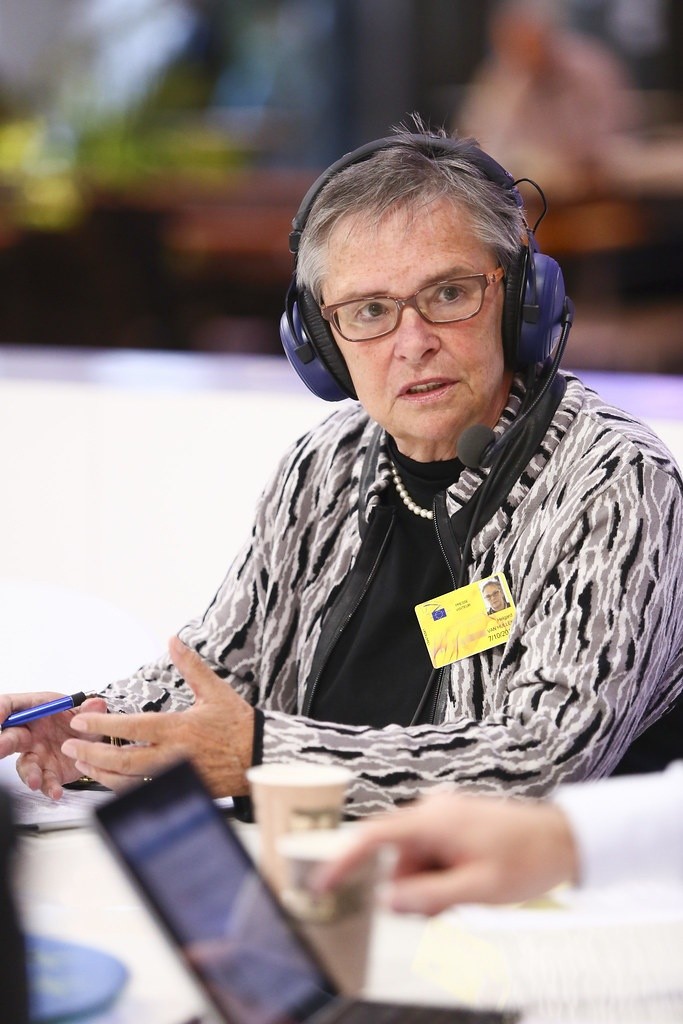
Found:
[1,765,683,1022]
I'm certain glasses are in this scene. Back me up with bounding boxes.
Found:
[324,265,508,343]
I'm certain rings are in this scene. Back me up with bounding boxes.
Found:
[142,776,155,787]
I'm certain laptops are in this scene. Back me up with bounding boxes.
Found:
[93,757,524,1024]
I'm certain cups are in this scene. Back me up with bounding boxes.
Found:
[245,760,353,889]
[274,832,369,957]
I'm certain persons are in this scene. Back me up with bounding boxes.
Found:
[304,739,683,924]
[448,3,643,197]
[0,120,683,832]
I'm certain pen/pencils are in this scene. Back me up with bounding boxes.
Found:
[3,691,86,728]
[17,819,88,833]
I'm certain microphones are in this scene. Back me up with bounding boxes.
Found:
[456,296,574,471]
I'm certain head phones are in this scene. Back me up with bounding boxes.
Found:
[280,134,569,403]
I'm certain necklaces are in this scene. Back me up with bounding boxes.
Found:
[376,430,479,523]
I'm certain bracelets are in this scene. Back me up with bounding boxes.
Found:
[74,719,118,783]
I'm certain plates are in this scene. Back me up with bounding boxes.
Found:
[25,930,127,1023]
